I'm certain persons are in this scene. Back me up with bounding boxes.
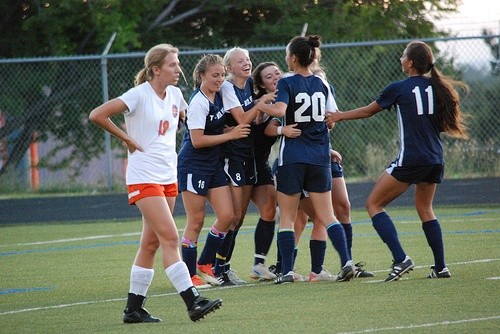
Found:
[220,37,373,287]
[89,43,222,323]
[178,53,250,290]
[324,41,465,286]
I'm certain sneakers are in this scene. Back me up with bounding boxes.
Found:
[249,263,276,279]
[215,264,247,284]
[354,261,374,277]
[191,275,212,288]
[425,266,450,278]
[269,265,276,274]
[195,269,225,286]
[335,260,355,282]
[122,307,162,324]
[273,271,294,284]
[309,265,338,282]
[384,255,414,282]
[188,297,222,322]
[293,273,309,281]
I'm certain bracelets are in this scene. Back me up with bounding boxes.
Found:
[277,126,283,135]
